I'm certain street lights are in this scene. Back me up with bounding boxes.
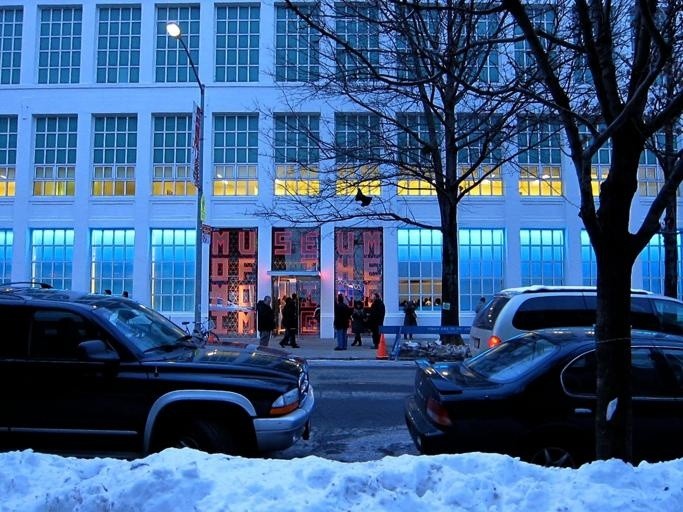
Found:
[164,21,206,341]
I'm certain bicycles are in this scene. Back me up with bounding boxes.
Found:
[179,317,221,345]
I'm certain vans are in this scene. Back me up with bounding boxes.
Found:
[471,283,683,356]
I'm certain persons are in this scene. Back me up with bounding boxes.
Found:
[475,297,485,316]
[272,290,441,346]
[368,293,385,350]
[257,295,276,347]
[278,293,300,348]
[103,289,112,295]
[334,294,350,350]
[116,291,129,325]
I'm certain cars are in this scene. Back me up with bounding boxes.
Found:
[0,283,315,457]
[404,327,683,464]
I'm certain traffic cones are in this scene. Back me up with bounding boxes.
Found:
[376,331,389,359]
